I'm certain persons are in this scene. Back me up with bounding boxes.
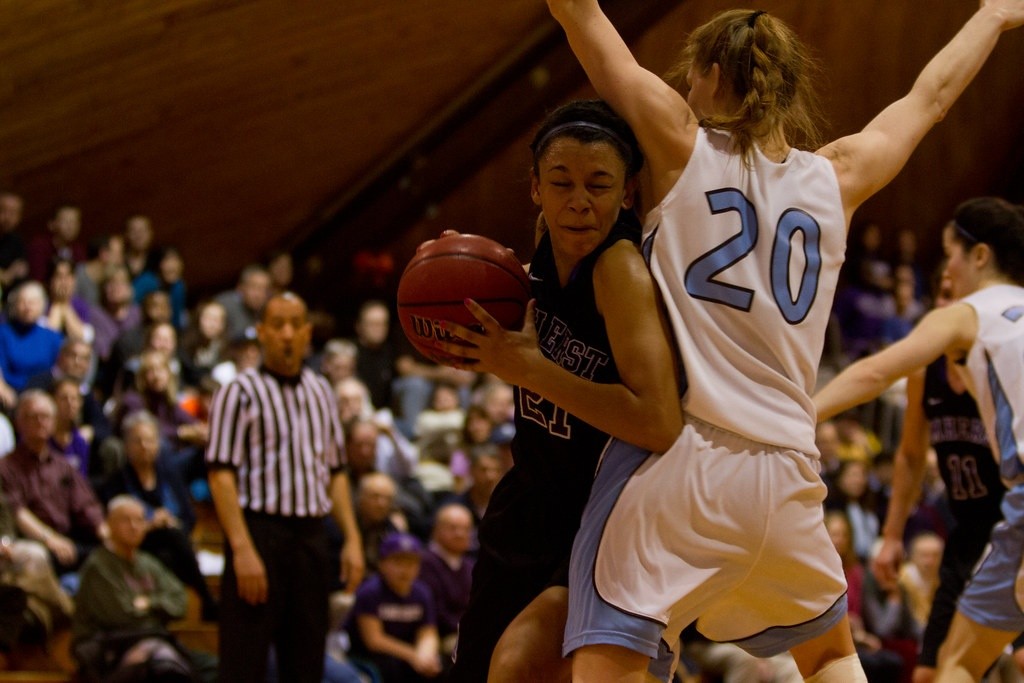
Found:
[415,100,684,683]
[0,176,1024,681]
[549,0,1024,683]
[208,292,364,682]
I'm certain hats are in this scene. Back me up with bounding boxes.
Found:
[378,532,422,557]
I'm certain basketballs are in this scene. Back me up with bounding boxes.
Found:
[395,233,536,368]
